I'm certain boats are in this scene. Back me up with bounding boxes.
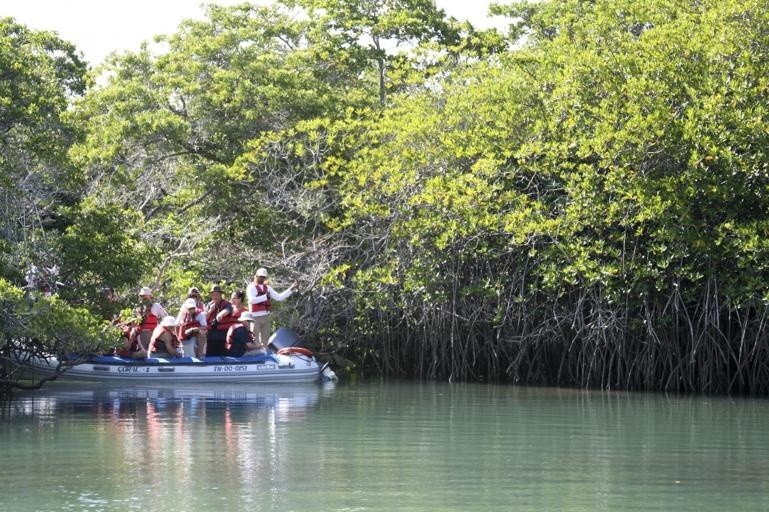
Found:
[0,332,339,384]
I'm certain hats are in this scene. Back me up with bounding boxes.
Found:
[160,316,179,327]
[238,311,256,322]
[209,285,226,295]
[256,268,268,277]
[138,287,153,297]
[184,298,197,309]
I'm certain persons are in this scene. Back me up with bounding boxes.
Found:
[26,262,298,360]
[98,395,293,491]
